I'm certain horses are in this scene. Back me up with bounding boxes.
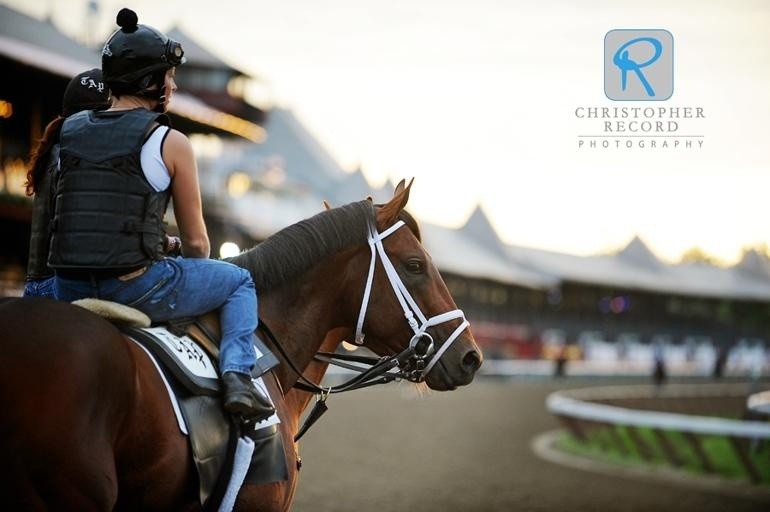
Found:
[0,175,484,512]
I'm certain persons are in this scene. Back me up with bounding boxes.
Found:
[22,68,112,300]
[46,8,277,422]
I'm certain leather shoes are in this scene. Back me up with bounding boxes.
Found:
[220,369,279,422]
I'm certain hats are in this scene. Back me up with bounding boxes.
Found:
[62,67,113,114]
[100,23,186,87]
